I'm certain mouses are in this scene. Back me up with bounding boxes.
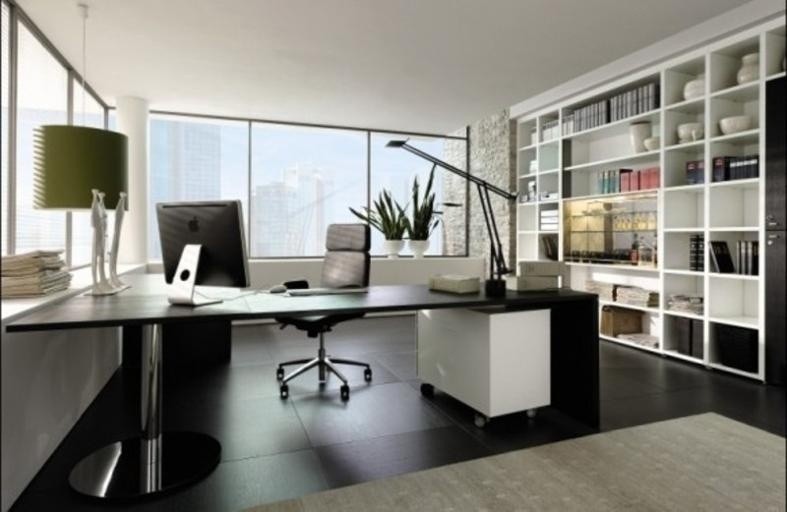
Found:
[269,285,287,293]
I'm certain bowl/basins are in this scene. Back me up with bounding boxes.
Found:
[716,116,753,136]
[642,137,661,150]
[681,79,704,99]
[675,121,704,139]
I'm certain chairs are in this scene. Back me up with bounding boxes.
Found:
[273,219,376,404]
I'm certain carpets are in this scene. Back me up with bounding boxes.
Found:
[240,410,787,510]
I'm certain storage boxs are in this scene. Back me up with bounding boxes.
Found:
[598,306,644,340]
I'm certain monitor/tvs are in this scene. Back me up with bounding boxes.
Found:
[156,200,251,306]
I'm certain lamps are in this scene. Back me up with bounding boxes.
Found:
[383,135,521,299]
[28,1,131,210]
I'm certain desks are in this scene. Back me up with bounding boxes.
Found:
[5,270,599,504]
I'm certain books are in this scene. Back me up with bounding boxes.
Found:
[597,165,661,195]
[1,248,71,299]
[709,238,759,276]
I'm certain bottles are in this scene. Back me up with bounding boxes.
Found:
[629,231,656,265]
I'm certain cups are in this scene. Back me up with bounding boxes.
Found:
[691,128,702,141]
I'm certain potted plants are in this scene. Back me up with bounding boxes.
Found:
[392,156,466,260]
[346,186,415,260]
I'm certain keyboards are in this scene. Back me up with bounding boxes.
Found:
[289,288,367,295]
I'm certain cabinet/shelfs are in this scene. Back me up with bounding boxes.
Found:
[506,11,785,386]
[413,304,556,430]
